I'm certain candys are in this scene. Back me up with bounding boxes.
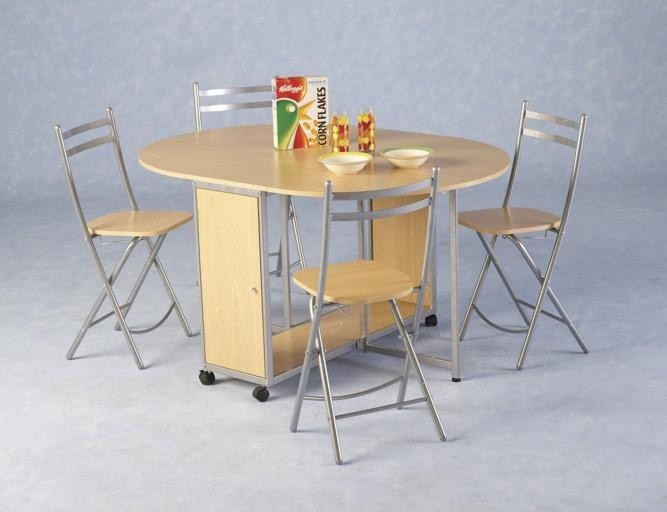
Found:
[332,112,375,154]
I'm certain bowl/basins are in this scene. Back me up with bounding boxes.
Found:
[382,146,433,170]
[317,152,373,175]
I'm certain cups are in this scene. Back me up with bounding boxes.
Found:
[331,108,351,154]
[357,105,376,156]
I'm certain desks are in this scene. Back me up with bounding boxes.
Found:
[135,122,511,402]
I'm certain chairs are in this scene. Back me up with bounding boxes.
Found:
[53,107,196,370]
[288,163,446,466]
[457,100,591,372]
[191,78,311,296]
